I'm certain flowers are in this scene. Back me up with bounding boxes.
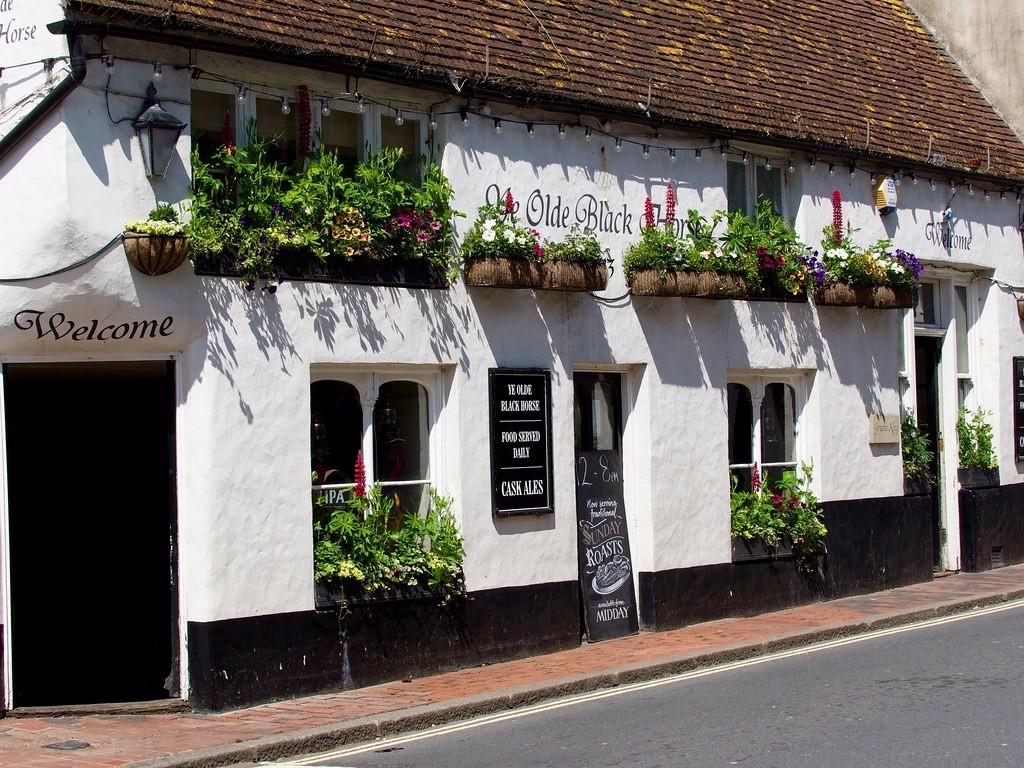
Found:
[957,406,999,470]
[726,457,829,581]
[239,132,324,294]
[328,151,394,262]
[480,218,543,259]
[867,239,924,285]
[541,222,607,264]
[395,163,460,286]
[310,449,475,607]
[820,190,873,284]
[124,219,184,236]
[623,181,724,271]
[727,194,826,295]
[901,405,933,483]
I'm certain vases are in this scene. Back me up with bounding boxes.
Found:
[392,257,431,288]
[270,248,303,279]
[904,474,933,495]
[957,466,1000,489]
[342,257,387,284]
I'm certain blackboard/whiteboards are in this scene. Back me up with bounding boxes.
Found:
[575,449,640,644]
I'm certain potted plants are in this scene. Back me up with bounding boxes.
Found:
[463,207,543,290]
[122,204,191,277]
[189,198,233,276]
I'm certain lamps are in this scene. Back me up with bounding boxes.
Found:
[132,82,188,179]
